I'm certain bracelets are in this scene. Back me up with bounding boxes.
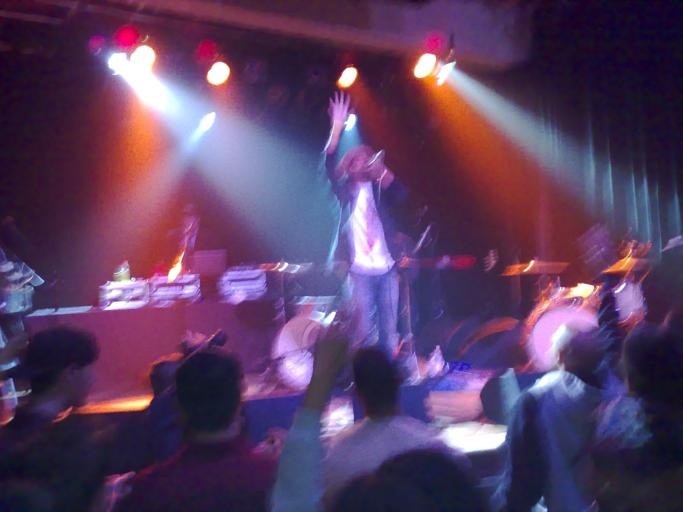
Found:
[376,169,388,183]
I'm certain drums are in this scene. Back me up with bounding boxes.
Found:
[522,290,593,369]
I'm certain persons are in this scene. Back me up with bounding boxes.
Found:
[0,233,682,510]
[323,92,409,359]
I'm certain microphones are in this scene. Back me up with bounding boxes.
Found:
[362,148,386,171]
[168,328,231,379]
